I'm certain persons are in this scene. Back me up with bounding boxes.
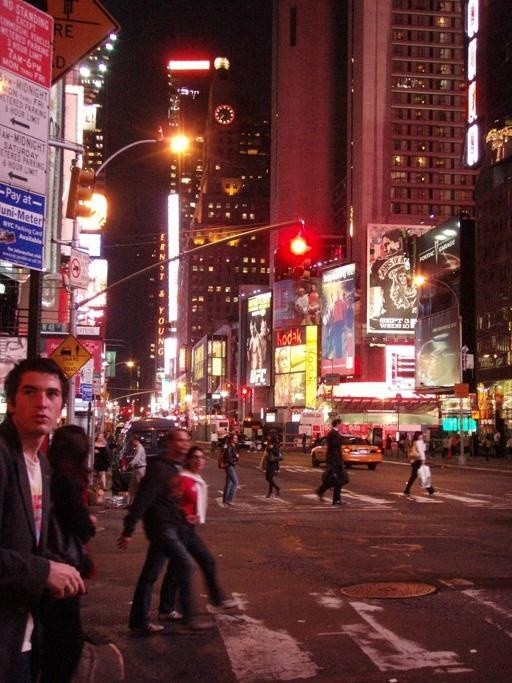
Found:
[159,446,237,620]
[211,431,218,448]
[223,433,239,506]
[324,289,354,361]
[404,431,439,496]
[1,359,86,683]
[301,434,306,452]
[125,434,147,505]
[371,230,418,327]
[263,430,282,497]
[47,424,98,643]
[117,430,215,631]
[94,432,111,489]
[248,318,272,385]
[386,427,512,465]
[275,348,290,373]
[316,419,347,506]
[292,271,322,325]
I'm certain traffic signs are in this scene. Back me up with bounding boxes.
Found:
[0,181,47,274]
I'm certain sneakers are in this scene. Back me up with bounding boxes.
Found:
[224,496,234,506]
[159,611,182,620]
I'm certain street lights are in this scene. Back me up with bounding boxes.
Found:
[94,133,192,177]
[66,219,308,425]
[412,274,467,464]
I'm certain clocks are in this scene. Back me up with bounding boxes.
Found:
[215,105,236,127]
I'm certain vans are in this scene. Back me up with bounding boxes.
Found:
[125,418,176,462]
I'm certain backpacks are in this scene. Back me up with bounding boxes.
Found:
[216,443,230,469]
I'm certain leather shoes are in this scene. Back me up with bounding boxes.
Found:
[266,487,281,498]
[146,620,164,631]
[316,488,342,504]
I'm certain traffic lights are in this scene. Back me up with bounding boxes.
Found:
[66,167,95,219]
[240,384,251,399]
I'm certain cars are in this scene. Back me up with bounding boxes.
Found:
[311,434,384,469]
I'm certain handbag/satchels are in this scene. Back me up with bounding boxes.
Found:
[416,462,431,488]
[328,463,349,487]
